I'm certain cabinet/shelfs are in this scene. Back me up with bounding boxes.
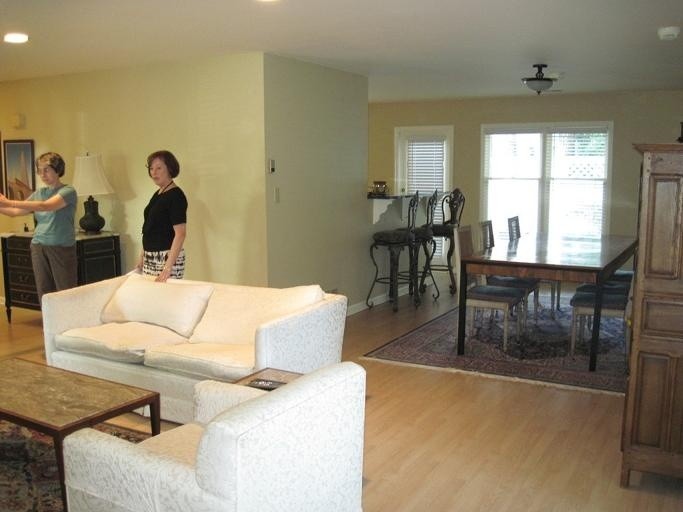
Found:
[620,143,681,491]
[2,234,120,324]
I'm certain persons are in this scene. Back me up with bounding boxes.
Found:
[137,151,188,281]
[1,152,77,301]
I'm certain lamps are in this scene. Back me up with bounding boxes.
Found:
[73,149,115,236]
[520,63,558,96]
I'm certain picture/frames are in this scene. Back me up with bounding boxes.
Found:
[2,139,36,201]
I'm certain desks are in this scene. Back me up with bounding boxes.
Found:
[368,194,436,304]
[457,235,639,371]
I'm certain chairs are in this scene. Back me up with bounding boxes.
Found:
[509,217,561,319]
[570,292,628,351]
[408,190,439,302]
[422,189,465,297]
[456,225,521,353]
[611,268,636,280]
[479,221,539,331]
[365,190,419,312]
[577,284,631,329]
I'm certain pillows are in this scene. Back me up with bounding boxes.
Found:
[101,275,212,338]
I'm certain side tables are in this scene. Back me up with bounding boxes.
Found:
[235,369,303,393]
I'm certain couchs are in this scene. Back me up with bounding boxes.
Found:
[41,270,347,425]
[64,362,367,511]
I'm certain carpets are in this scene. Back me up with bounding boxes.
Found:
[361,306,629,395]
[0,422,152,512]
